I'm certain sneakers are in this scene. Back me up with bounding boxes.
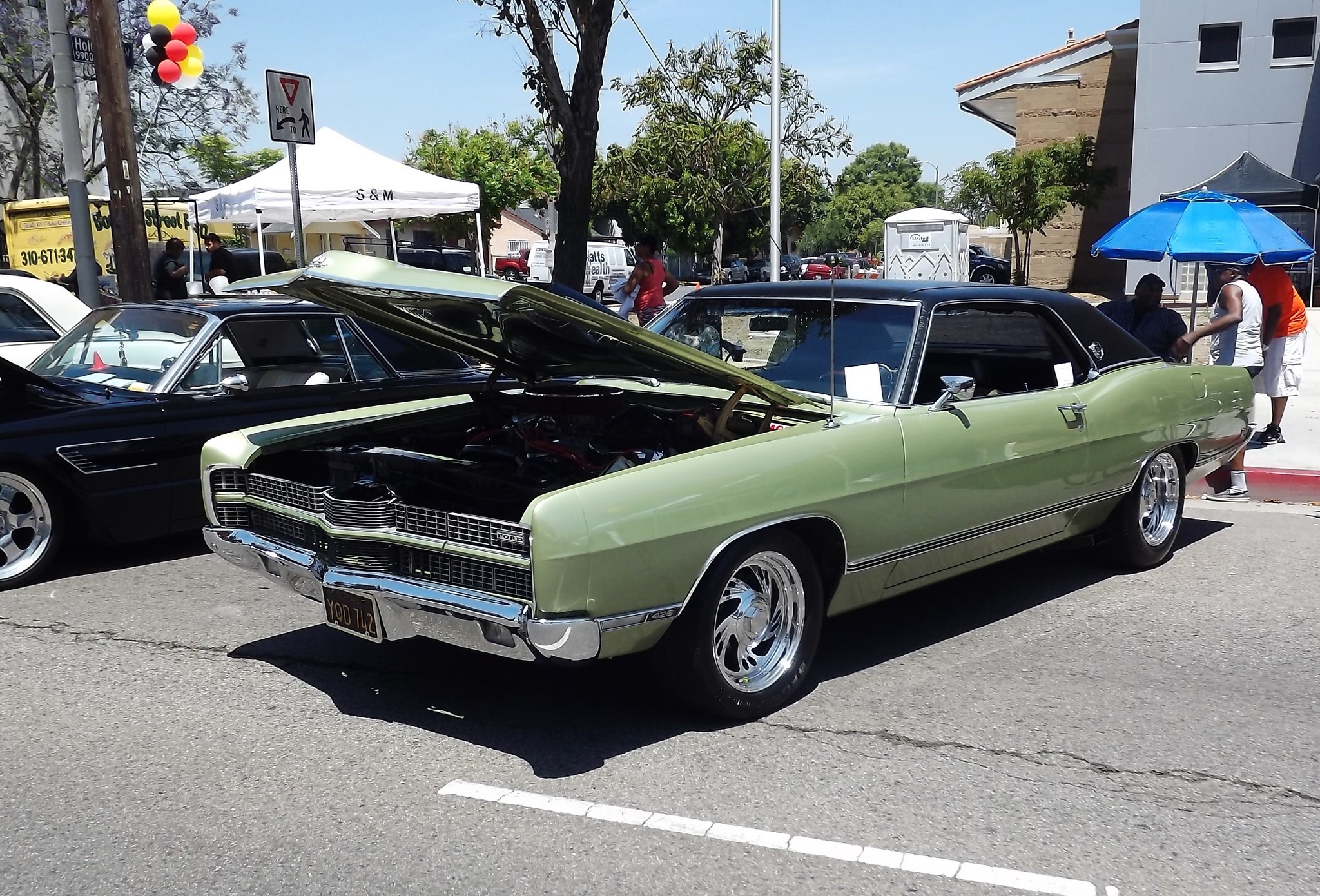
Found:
[1257,424,1285,445]
[1202,489,1250,501]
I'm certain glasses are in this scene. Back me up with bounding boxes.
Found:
[1215,268,1234,275]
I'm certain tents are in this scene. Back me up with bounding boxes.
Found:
[1159,151,1320,309]
[189,126,484,284]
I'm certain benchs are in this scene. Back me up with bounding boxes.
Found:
[866,349,1054,400]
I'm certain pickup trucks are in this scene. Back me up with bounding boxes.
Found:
[493,248,531,282]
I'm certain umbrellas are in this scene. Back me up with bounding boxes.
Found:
[1092,187,1316,366]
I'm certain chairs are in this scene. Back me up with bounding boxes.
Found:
[947,350,998,397]
[188,363,329,390]
[783,344,832,381]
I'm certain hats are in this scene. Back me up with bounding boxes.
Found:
[1222,264,1253,274]
[1138,274,1165,289]
[201,233,221,242]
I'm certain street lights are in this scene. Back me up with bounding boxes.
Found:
[913,161,939,210]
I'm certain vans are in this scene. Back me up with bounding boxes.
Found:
[174,247,298,294]
[388,245,482,277]
[527,240,642,304]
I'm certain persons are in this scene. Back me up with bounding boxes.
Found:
[1175,259,1308,503]
[199,233,239,294]
[1096,274,1189,363]
[55,255,103,303]
[154,238,189,298]
[624,232,679,328]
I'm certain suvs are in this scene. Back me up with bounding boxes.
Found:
[779,254,802,281]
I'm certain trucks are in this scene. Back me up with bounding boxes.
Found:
[2,193,252,301]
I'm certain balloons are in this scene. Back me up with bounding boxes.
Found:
[142,0,204,90]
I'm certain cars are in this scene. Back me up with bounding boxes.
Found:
[743,259,770,282]
[694,257,750,285]
[969,243,1011,285]
[1,295,513,592]
[798,251,885,281]
[0,269,246,392]
[199,250,1256,724]
